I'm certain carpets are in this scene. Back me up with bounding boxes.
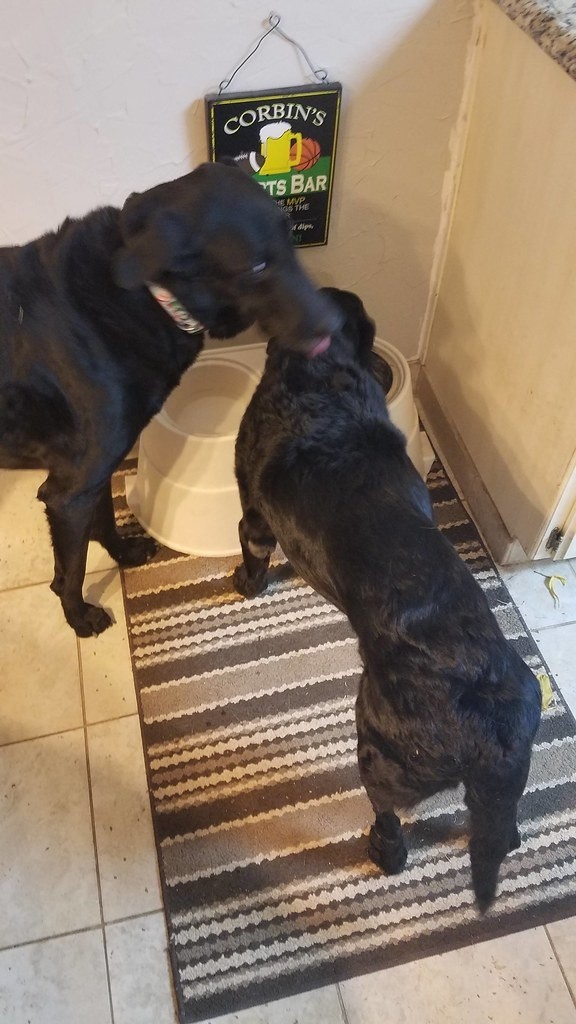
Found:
[108,412,576,1024]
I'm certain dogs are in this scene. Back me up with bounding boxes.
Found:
[1,162,344,639]
[235,286,544,920]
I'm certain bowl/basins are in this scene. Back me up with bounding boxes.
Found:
[158,358,270,443]
[361,351,396,399]
